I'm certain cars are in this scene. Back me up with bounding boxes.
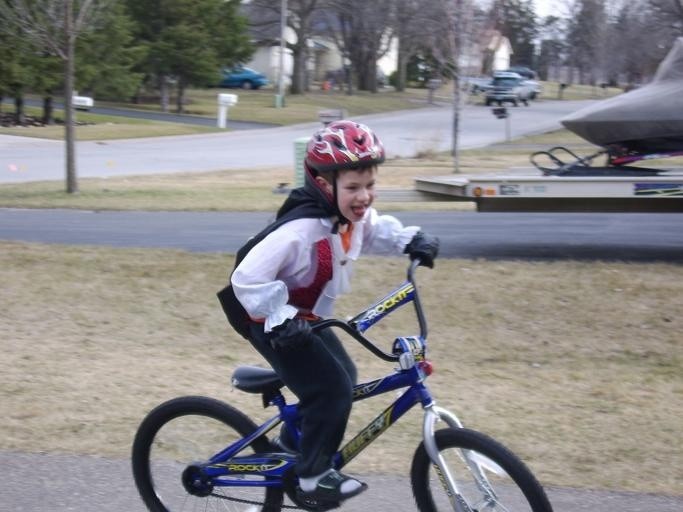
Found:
[198,60,266,90]
[323,62,387,89]
[388,63,441,90]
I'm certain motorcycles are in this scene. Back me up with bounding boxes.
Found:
[527,35,683,179]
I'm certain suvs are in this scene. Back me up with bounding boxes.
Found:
[480,72,529,108]
[468,67,541,99]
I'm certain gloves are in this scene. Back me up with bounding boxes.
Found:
[266,317,313,353]
[405,230,440,271]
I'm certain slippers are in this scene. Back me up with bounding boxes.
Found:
[292,471,369,502]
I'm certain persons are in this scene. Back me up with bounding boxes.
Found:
[217,118,440,504]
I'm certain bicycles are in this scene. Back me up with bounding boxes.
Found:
[129,249,554,512]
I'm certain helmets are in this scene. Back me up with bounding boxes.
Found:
[303,120,385,173]
